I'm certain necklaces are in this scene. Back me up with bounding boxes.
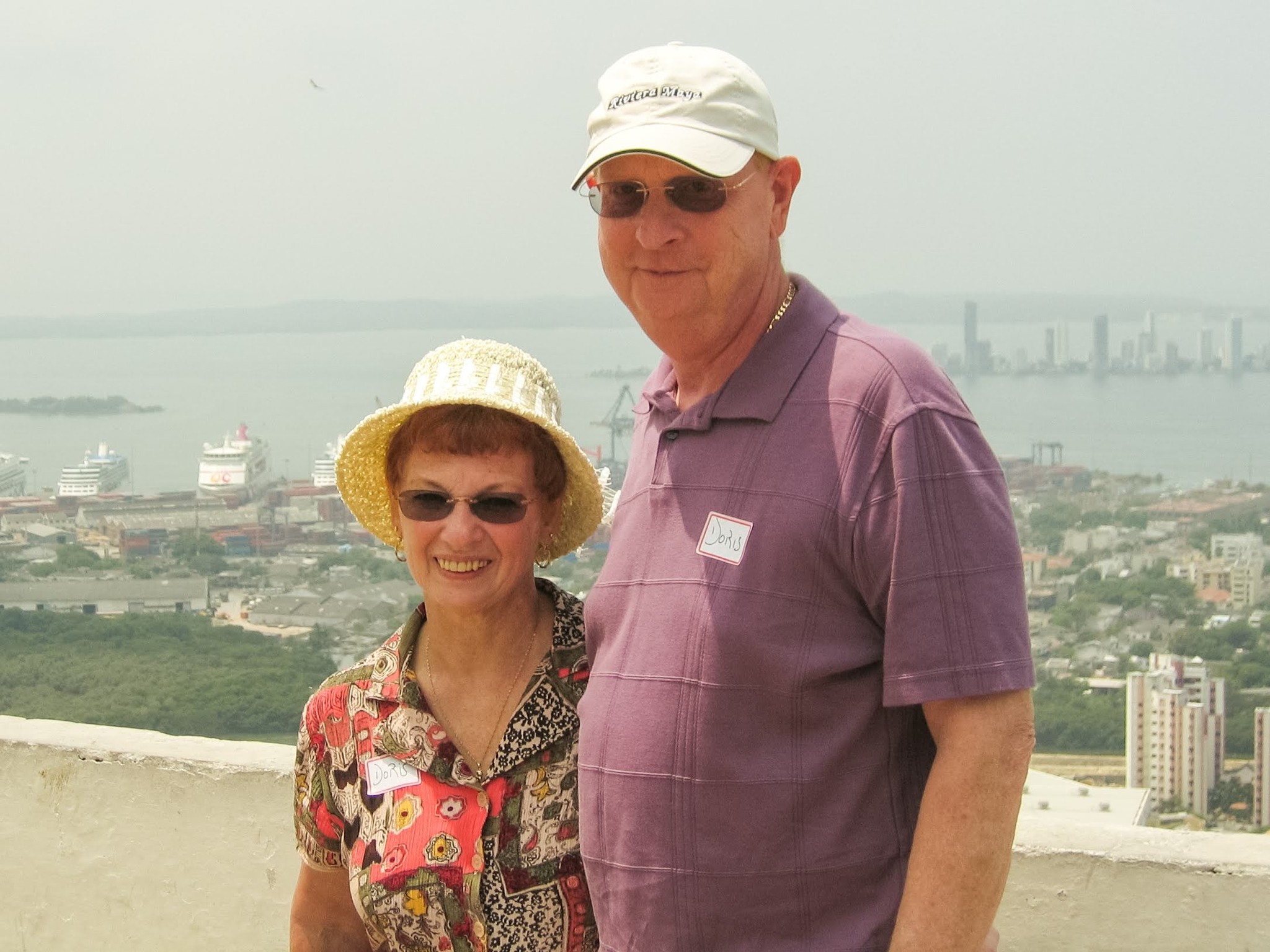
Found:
[765,282,797,336]
[426,600,540,782]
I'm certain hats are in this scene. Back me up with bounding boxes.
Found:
[333,338,604,562]
[571,41,781,191]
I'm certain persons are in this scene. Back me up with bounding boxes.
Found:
[288,338,599,952]
[572,41,1036,952]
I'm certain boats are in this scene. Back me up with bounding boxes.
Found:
[311,433,346,489]
[0,452,31,498]
[196,423,276,505]
[53,439,131,509]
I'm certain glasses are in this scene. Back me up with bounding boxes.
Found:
[587,158,769,219]
[391,474,546,524]
